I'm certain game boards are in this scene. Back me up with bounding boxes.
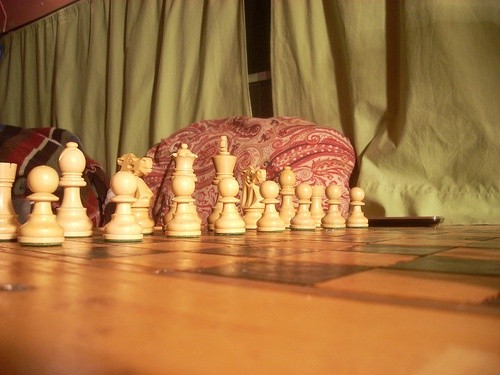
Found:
[0,224,500,321]
